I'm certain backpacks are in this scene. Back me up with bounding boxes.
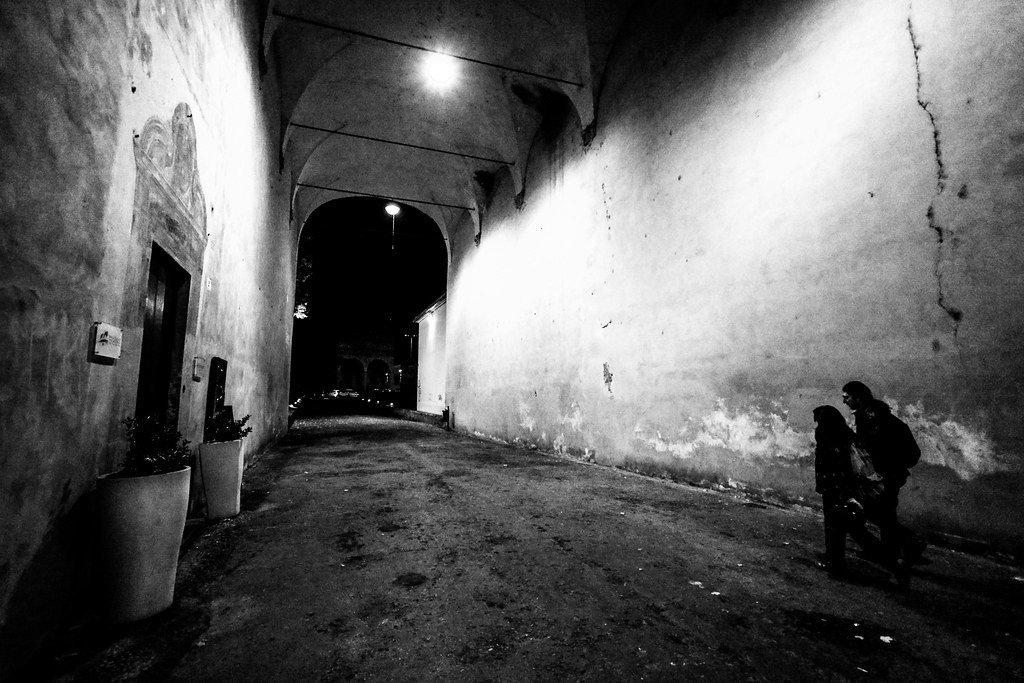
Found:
[833,437,887,516]
[882,412,922,469]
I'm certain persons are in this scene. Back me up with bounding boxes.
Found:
[812,405,913,587]
[842,380,927,560]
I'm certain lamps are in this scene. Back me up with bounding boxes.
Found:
[384,200,401,216]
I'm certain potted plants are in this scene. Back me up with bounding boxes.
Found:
[94,412,192,622]
[192,407,252,519]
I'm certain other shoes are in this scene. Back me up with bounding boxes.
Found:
[904,535,927,566]
[897,563,913,590]
[814,559,843,573]
